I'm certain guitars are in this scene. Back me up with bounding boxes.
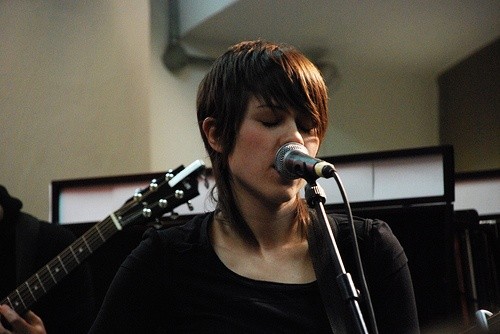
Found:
[1,158,210,316]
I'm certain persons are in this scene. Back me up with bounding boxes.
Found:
[0,184,95,334]
[88,39,421,334]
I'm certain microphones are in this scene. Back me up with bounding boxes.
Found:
[275,142,336,179]
